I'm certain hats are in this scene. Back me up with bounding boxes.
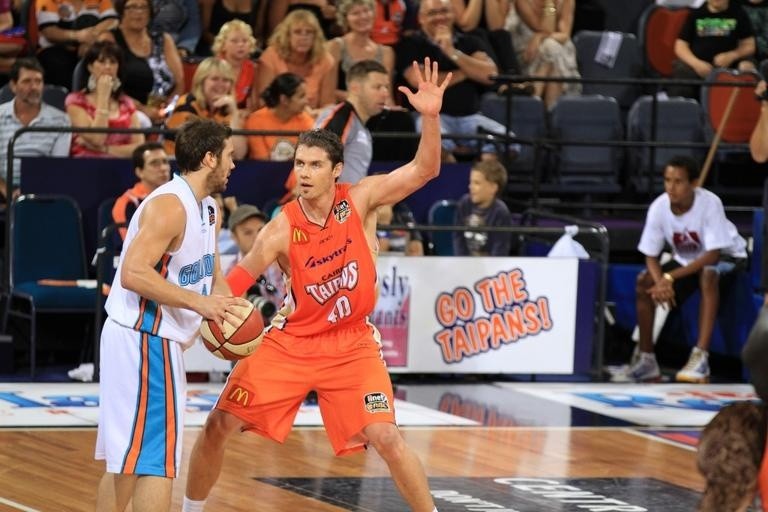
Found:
[228,203,269,230]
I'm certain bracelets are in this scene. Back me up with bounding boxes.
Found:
[663,273,674,283]
[450,50,463,61]
[97,110,109,116]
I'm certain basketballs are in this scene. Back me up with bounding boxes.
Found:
[200,298,264,360]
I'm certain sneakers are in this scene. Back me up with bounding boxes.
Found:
[675,346,710,383]
[610,351,660,383]
[68,363,95,382]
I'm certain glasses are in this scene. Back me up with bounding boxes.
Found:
[126,3,148,12]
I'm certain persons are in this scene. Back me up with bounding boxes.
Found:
[64,39,146,159]
[451,0,536,97]
[674,0,756,102]
[270,0,337,40]
[160,55,248,160]
[149,0,203,64]
[249,9,337,114]
[102,141,172,296]
[211,19,262,108]
[328,0,397,107]
[214,204,287,312]
[391,0,523,166]
[372,172,424,256]
[93,115,252,512]
[201,0,266,59]
[609,153,749,384]
[370,0,408,46]
[208,192,240,255]
[243,72,314,162]
[0,57,73,213]
[98,0,185,130]
[503,0,584,111]
[450,159,511,256]
[35,0,119,91]
[742,0,768,103]
[0,0,31,67]
[180,56,454,512]
[309,59,391,185]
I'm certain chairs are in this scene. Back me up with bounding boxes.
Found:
[627,91,713,198]
[569,28,643,99]
[480,93,549,181]
[95,195,124,287]
[548,95,623,197]
[634,3,700,80]
[4,193,109,379]
[700,67,766,166]
[427,196,461,254]
